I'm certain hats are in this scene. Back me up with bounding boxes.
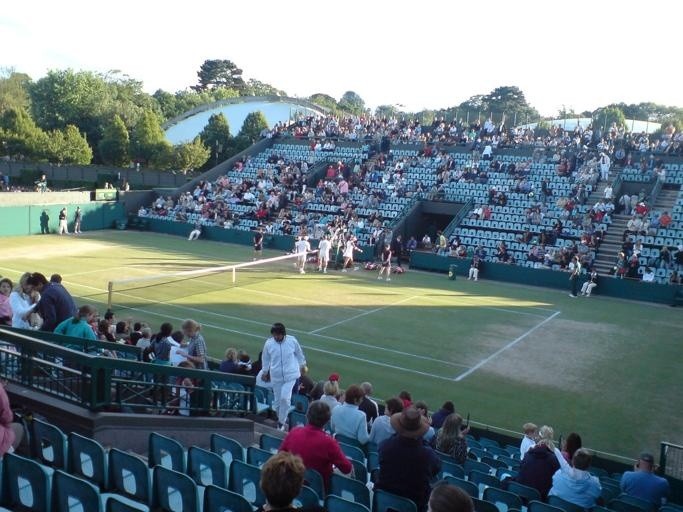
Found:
[390,407,429,439]
[639,452,654,462]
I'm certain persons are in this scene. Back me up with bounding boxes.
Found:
[124,180,130,192]
[0,271,308,406]
[1,376,25,460]
[261,322,309,432]
[135,109,683,308]
[426,481,477,511]
[277,364,682,512]
[255,450,309,512]
[58,207,71,235]
[35,173,52,192]
[73,206,83,234]
[39,209,51,234]
